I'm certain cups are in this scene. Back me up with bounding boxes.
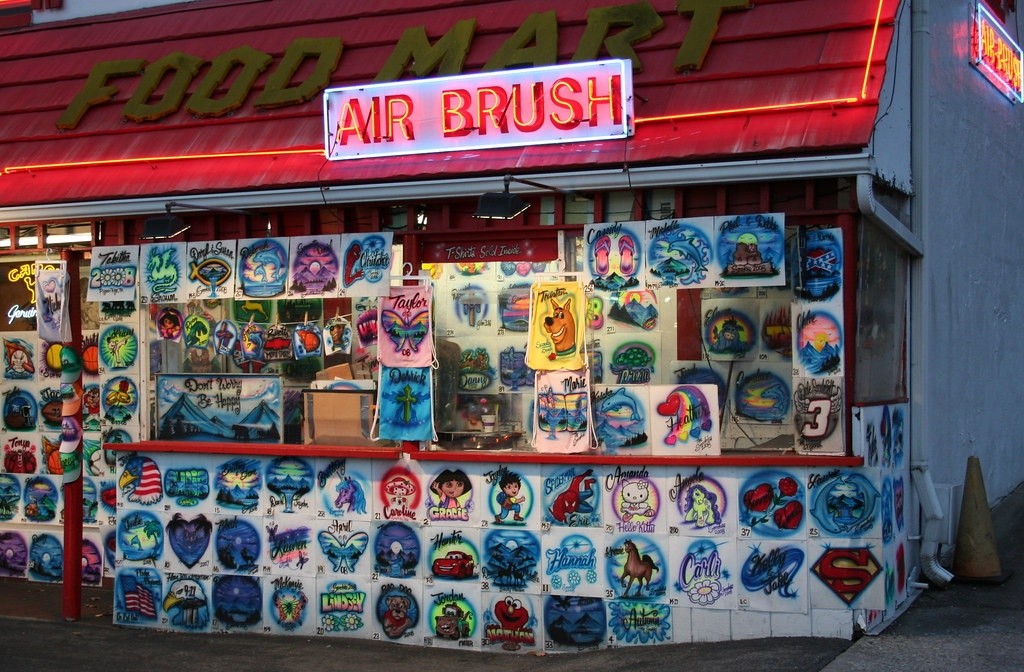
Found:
[482,415,494,432]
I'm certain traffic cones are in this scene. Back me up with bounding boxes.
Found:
[946,455,1015,588]
